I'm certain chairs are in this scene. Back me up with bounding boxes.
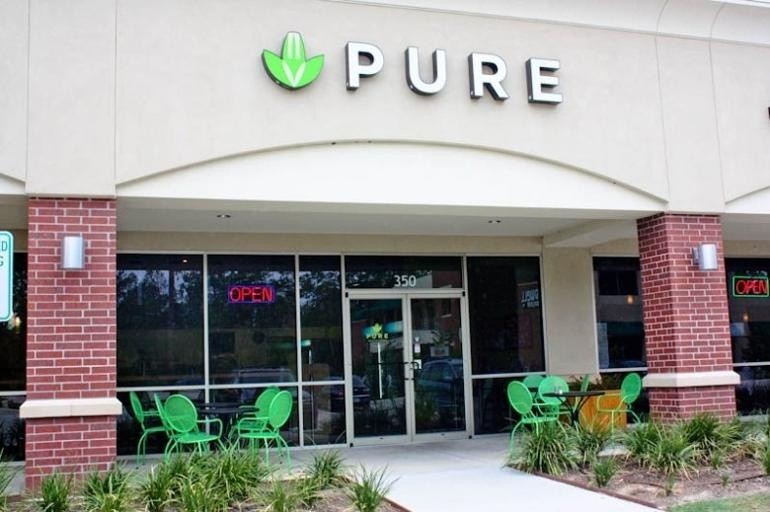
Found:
[126,385,293,475]
[505,372,642,462]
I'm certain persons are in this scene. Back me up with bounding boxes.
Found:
[141,336,238,402]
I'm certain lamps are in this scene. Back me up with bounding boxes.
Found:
[692,243,718,272]
[60,235,84,270]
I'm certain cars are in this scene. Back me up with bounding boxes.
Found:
[735,368,770,396]
[230,368,318,430]
[415,358,497,408]
[316,375,371,412]
[608,359,646,368]
